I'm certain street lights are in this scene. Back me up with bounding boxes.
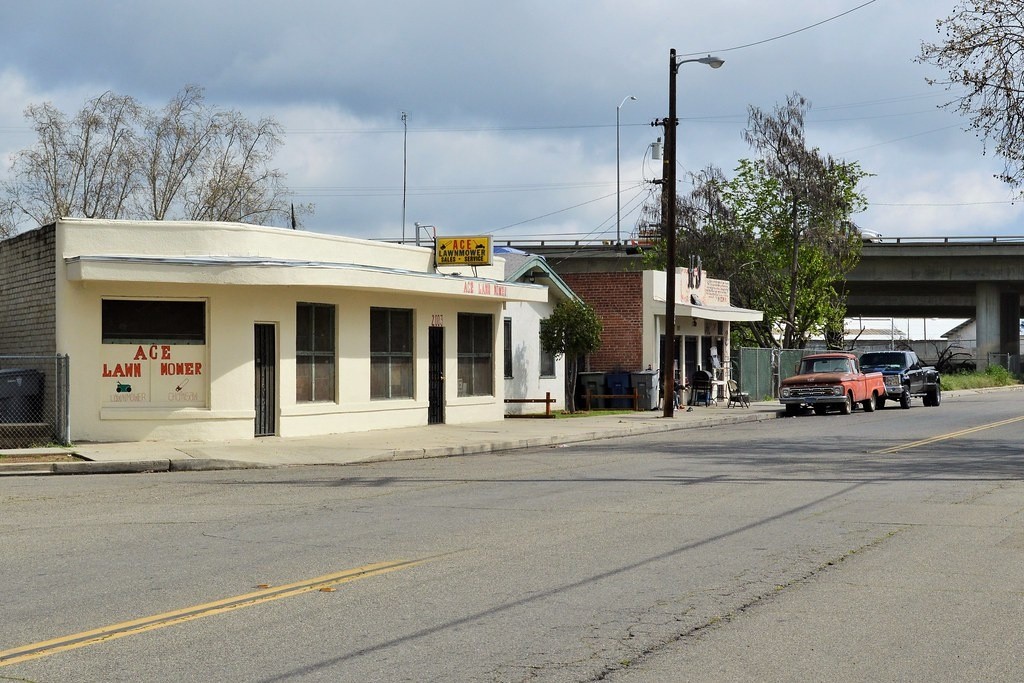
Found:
[616,95,638,241]
[402,113,409,244]
[664,56,725,412]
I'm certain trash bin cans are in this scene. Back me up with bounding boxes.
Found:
[604,372,633,411]
[0,367,43,436]
[631,371,660,410]
[578,371,604,411]
[1010,355,1024,385]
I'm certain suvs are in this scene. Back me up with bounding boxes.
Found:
[834,219,882,244]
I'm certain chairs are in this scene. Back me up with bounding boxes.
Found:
[727,379,750,409]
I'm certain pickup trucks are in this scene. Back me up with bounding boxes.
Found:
[779,352,886,414]
[859,350,942,407]
